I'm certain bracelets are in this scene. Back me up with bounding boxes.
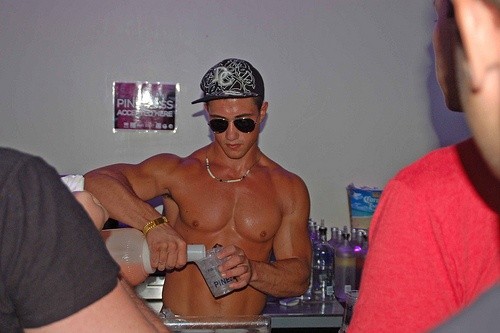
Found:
[144,217,168,233]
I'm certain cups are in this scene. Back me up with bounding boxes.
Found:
[195,247,238,298]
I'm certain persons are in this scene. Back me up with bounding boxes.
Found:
[343,0,500,333]
[0,145,172,333]
[429,0,500,333]
[83,57,314,329]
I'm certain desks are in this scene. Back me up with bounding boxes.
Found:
[261,300,345,333]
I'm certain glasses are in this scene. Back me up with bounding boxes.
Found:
[207,105,263,134]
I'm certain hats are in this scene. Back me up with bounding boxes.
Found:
[191,58,264,104]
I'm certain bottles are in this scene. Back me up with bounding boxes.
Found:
[100,228,206,286]
[278,219,368,333]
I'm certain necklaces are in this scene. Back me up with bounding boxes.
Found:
[205,147,251,183]
